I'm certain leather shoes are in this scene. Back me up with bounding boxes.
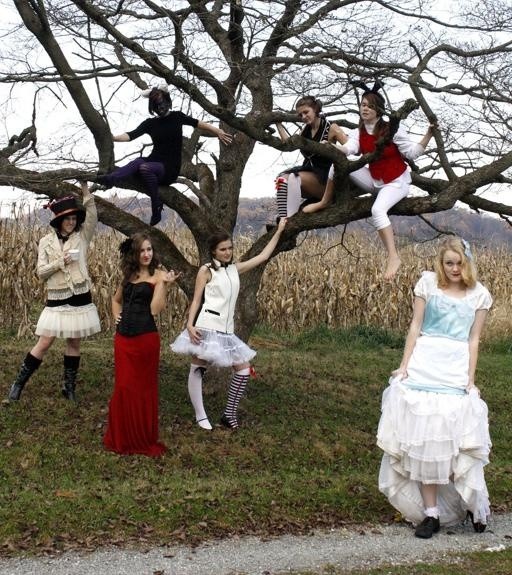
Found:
[415,517,440,539]
[467,510,486,533]
[220,414,233,429]
[150,203,164,226]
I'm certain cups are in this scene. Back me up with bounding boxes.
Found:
[67,250,80,261]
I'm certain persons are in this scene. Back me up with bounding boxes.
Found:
[274,95,349,224]
[102,232,182,458]
[168,217,288,431]
[98,84,233,226]
[376,236,493,538]
[9,181,102,403]
[302,91,441,281]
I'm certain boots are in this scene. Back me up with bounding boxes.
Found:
[8,352,42,400]
[62,356,81,400]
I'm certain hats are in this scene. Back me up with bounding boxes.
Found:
[50,196,85,229]
